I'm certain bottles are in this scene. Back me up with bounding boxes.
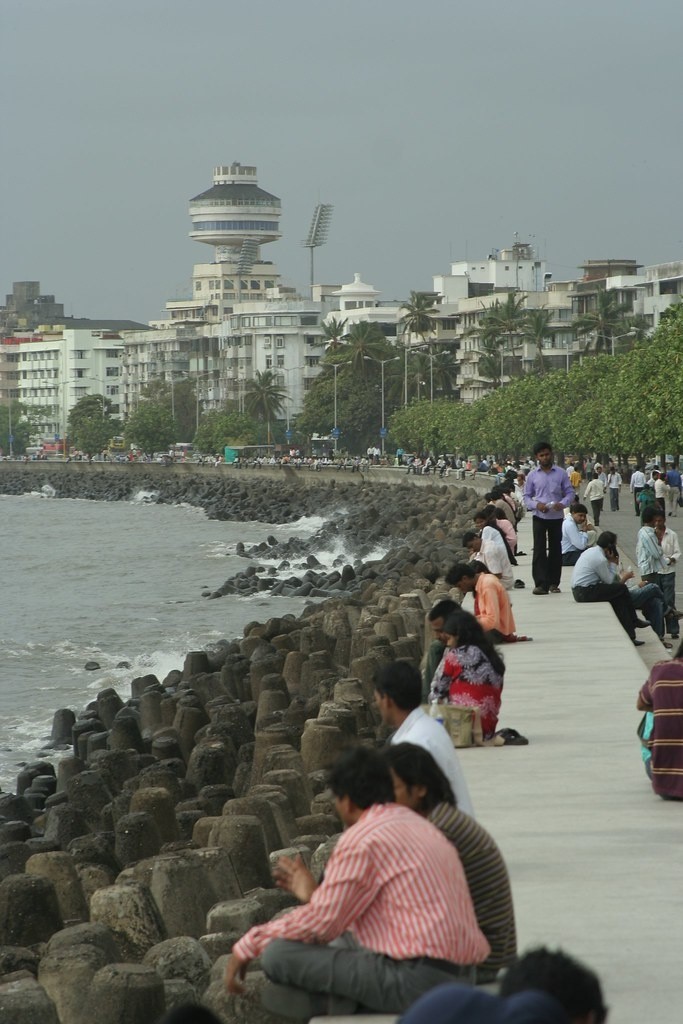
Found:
[619,562,623,570]
[626,566,633,588]
[429,700,443,725]
[545,501,554,509]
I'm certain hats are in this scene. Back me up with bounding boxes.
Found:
[394,981,557,1024]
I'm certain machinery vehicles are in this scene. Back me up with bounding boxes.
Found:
[102,435,128,461]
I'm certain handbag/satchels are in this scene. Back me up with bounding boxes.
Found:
[679,496,683,507]
[423,702,503,748]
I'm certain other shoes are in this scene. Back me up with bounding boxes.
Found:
[668,512,673,516]
[662,640,673,648]
[665,609,683,620]
[636,619,651,628]
[532,586,548,594]
[514,579,525,588]
[632,638,645,646]
[516,551,526,556]
[548,585,561,593]
[671,634,679,639]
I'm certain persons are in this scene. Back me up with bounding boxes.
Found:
[370,663,472,819]
[225,747,491,1018]
[637,648,683,800]
[398,947,608,1024]
[381,742,517,985]
[3,441,683,647]
[164,1001,219,1024]
[422,600,530,749]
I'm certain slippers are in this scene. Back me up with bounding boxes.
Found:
[488,728,527,745]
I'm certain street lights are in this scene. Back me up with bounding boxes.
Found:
[0,383,22,456]
[404,344,428,409]
[318,360,353,448]
[227,369,258,413]
[42,380,81,458]
[89,376,119,419]
[363,355,400,455]
[270,365,307,444]
[562,340,570,375]
[588,331,636,356]
[182,371,213,432]
[412,351,451,403]
[481,345,523,387]
